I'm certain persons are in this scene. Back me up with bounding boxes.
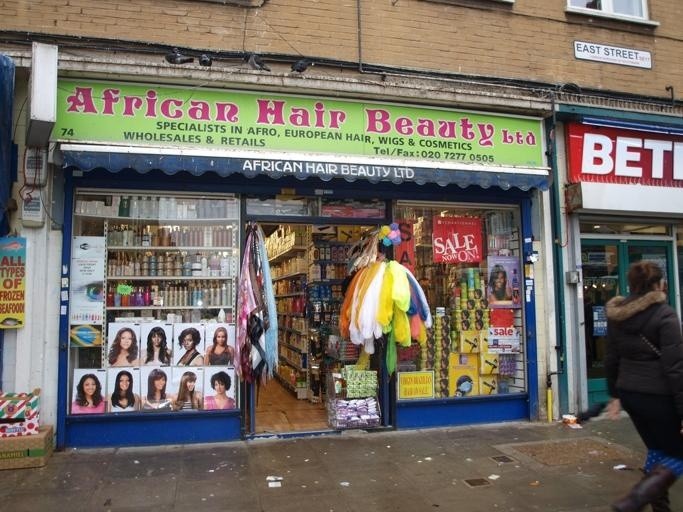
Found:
[488,263,512,303]
[202,324,233,366]
[600,260,682,512]
[72,373,105,413]
[174,326,200,367]
[140,327,171,369]
[107,326,138,368]
[204,371,235,410]
[106,370,140,413]
[142,368,170,412]
[172,371,200,411]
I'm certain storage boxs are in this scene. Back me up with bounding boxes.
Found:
[458,309,514,396]
[0,391,41,438]
[0,424,55,472]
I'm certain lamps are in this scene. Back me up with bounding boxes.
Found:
[164,49,310,73]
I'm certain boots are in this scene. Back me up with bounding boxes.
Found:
[610,461,679,512]
[650,489,673,512]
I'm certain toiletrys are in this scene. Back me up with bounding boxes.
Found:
[75,196,239,324]
[270,244,360,389]
